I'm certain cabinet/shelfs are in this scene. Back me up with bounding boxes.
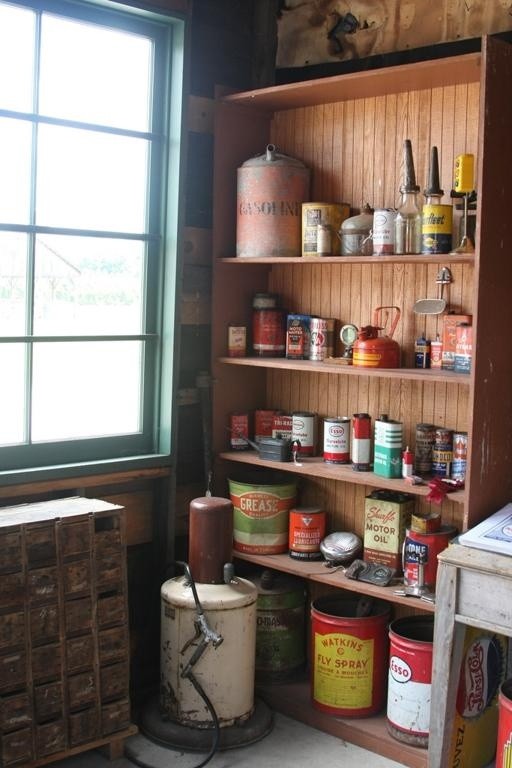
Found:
[0,491,139,766]
[204,32,511,767]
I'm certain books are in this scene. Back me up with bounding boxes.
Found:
[452,502,512,558]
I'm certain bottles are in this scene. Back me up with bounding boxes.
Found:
[454,320,472,373]
[420,190,444,205]
[393,187,421,255]
[316,224,333,257]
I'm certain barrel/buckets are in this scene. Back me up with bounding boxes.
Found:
[493,678,512,767]
[246,572,310,688]
[299,199,352,259]
[402,521,456,595]
[383,614,434,750]
[308,590,394,722]
[227,469,299,556]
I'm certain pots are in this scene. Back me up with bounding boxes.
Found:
[337,205,374,255]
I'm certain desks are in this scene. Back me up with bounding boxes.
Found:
[424,543,510,768]
